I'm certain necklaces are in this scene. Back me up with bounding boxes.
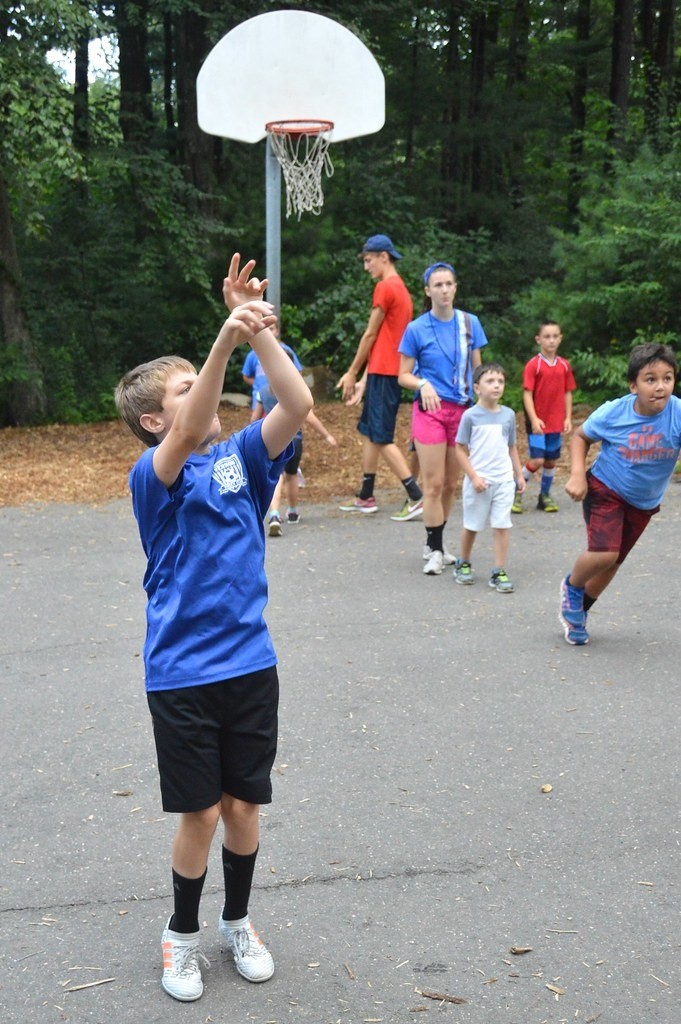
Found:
[429,310,457,385]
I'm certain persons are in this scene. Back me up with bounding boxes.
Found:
[510,318,576,515]
[453,362,526,593]
[242,318,307,489]
[397,262,489,575]
[335,234,424,521]
[250,349,337,536]
[113,253,314,1005]
[557,342,681,645]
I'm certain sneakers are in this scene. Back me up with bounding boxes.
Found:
[269,516,284,537]
[390,496,424,522]
[423,545,457,564]
[161,913,211,1002]
[287,510,302,524]
[558,611,590,645]
[558,573,587,627]
[423,549,445,575]
[537,494,560,512]
[488,568,516,593]
[453,558,476,585]
[512,494,524,513]
[337,495,379,513]
[220,915,274,983]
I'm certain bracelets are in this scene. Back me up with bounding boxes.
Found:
[417,378,427,390]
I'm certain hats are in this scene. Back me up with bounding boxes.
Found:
[362,234,402,260]
[423,262,456,284]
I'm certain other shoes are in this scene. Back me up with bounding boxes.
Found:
[297,467,307,488]
